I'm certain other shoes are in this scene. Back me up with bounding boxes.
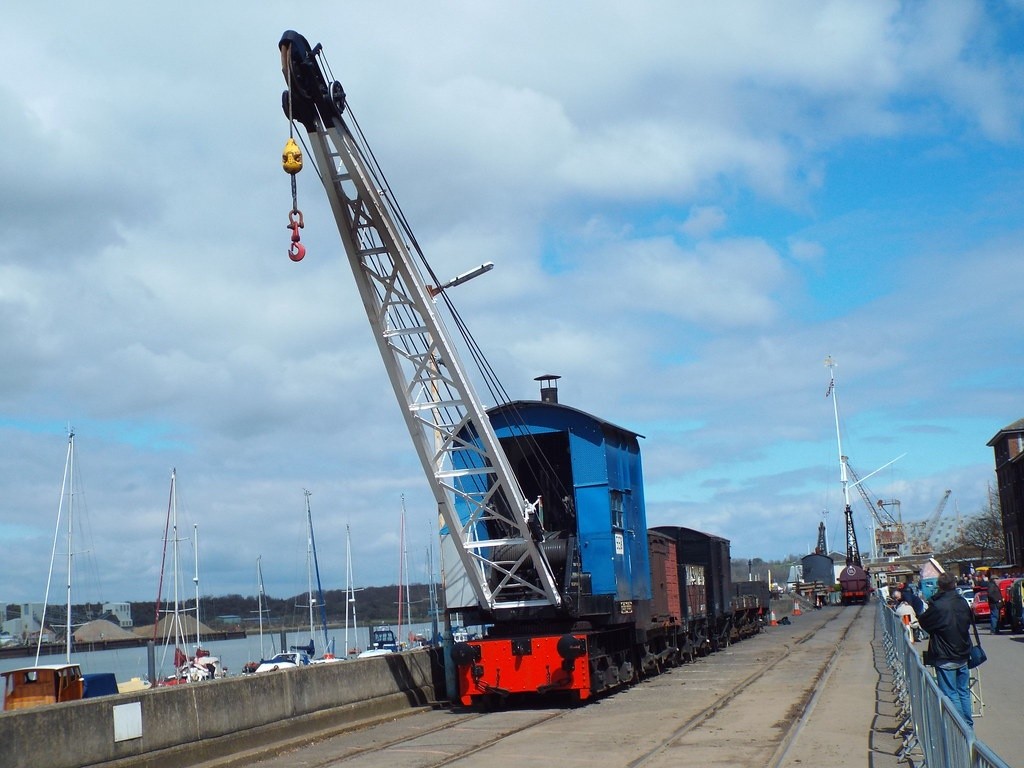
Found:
[914,639,921,642]
[990,631,1003,635]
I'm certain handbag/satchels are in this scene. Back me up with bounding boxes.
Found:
[967,645,988,670]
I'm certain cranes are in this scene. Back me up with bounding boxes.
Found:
[909,490,952,555]
[841,456,907,557]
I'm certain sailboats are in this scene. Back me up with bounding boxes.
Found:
[0,419,476,711]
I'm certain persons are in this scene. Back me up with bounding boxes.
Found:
[955,573,1024,587]
[899,581,914,595]
[895,601,922,642]
[987,575,1005,635]
[887,590,929,640]
[918,573,974,730]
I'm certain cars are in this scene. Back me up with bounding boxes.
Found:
[956,578,1024,633]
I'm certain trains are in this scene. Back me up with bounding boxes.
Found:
[825,564,872,606]
[436,374,772,714]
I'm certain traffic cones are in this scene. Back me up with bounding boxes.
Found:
[791,598,802,615]
[769,609,778,626]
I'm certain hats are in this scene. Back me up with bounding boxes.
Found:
[991,575,1000,579]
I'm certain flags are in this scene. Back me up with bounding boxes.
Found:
[826,379,834,397]
